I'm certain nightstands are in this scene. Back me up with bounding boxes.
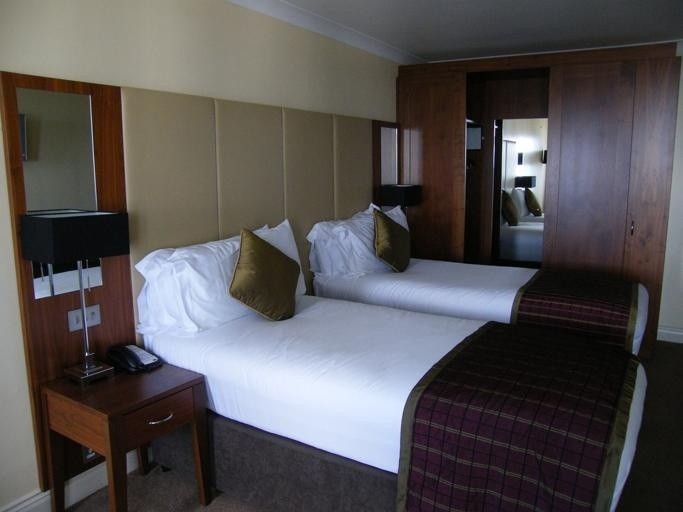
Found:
[36,360,215,511]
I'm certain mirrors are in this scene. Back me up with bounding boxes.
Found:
[16,86,98,217]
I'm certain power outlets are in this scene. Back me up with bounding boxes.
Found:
[67,304,102,332]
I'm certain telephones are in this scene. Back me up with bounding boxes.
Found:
[106,343,163,374]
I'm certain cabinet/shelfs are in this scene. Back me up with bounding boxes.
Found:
[396,44,680,357]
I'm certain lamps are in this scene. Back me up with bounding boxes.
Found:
[20,210,130,389]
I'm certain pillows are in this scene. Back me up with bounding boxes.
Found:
[332,204,411,280]
[134,223,270,339]
[372,208,411,273]
[306,201,380,281]
[227,228,301,324]
[162,219,310,339]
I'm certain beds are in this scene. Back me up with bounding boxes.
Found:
[312,256,651,354]
[144,293,649,510]
